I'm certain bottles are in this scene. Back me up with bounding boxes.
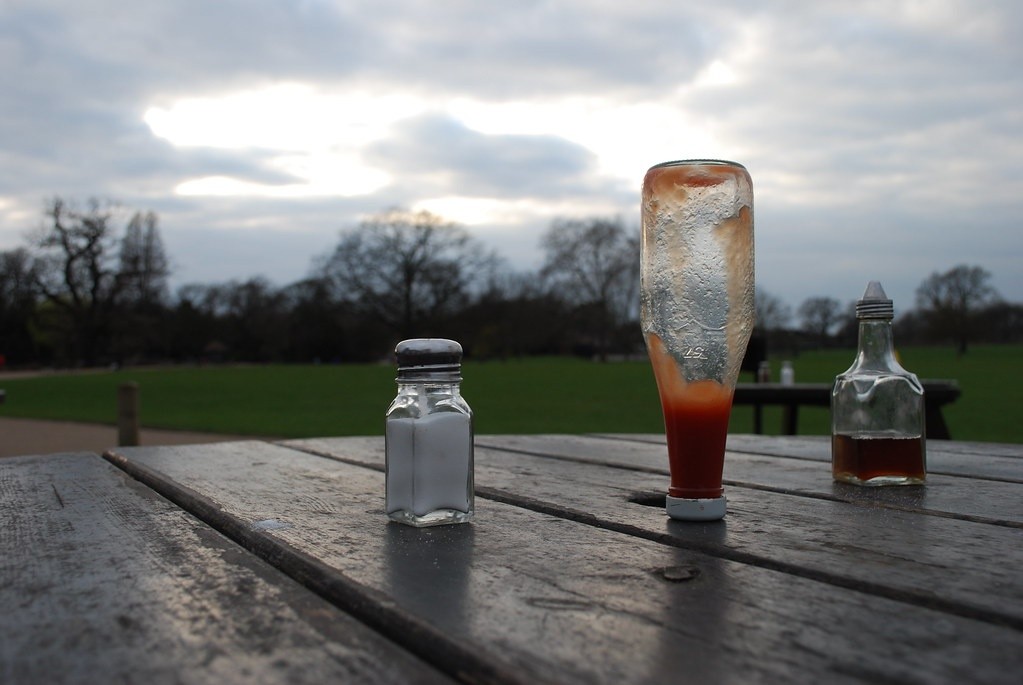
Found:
[384,338,474,527]
[640,158,755,520]
[779,361,795,384]
[831,280,927,486]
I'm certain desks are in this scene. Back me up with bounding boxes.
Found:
[0,434,1023,685]
[732,379,961,441]
